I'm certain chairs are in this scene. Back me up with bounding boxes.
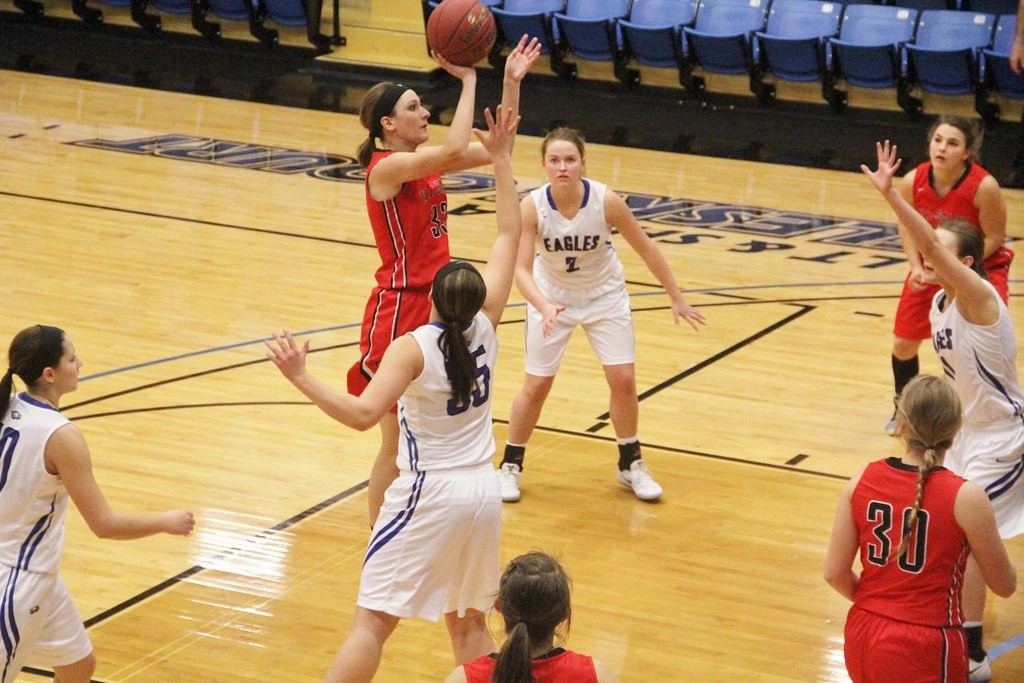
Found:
[11,0,335,53]
[488,0,567,72]
[748,0,842,105]
[610,0,698,90]
[894,7,997,127]
[975,13,1024,136]
[548,0,631,80]
[675,0,770,100]
[819,3,920,119]
[425,0,503,8]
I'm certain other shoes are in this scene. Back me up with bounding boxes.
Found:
[969,655,992,683]
[886,398,898,435]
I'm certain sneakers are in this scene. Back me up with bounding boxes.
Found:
[617,459,663,500]
[496,462,522,502]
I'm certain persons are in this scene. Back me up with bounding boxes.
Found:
[861,140,1024,683]
[822,376,1018,683]
[347,35,543,531]
[1009,0,1024,72]
[265,106,522,683]
[886,114,1015,435]
[0,324,195,683]
[499,127,708,502]
[444,552,615,683]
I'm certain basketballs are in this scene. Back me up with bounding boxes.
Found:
[425,0,497,67]
